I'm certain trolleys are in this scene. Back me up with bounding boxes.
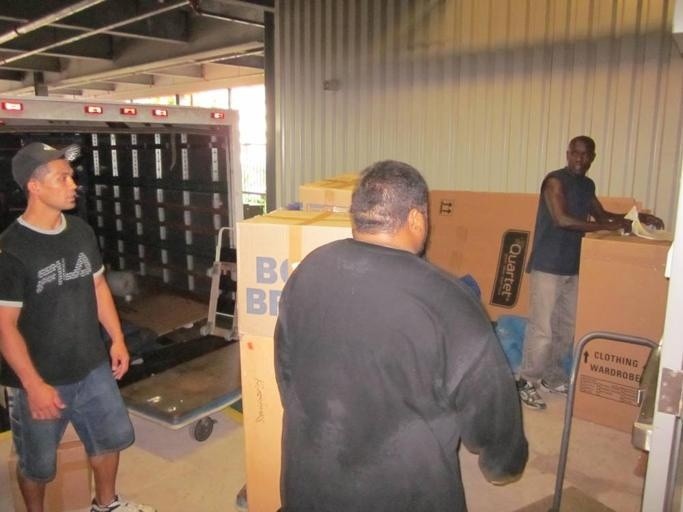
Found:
[115,294,208,340]
[119,226,241,442]
[513,331,657,512]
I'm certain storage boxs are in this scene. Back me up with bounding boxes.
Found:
[299,173,361,212]
[236,208,353,512]
[424,190,635,322]
[571,231,674,433]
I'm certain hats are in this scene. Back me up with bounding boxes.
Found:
[12,142,80,190]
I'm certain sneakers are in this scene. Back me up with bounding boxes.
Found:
[90,495,156,512]
[541,379,568,395]
[516,379,546,409]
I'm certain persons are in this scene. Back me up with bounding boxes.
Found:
[0,141,158,511]
[514,136,665,410]
[273,160,529,512]
